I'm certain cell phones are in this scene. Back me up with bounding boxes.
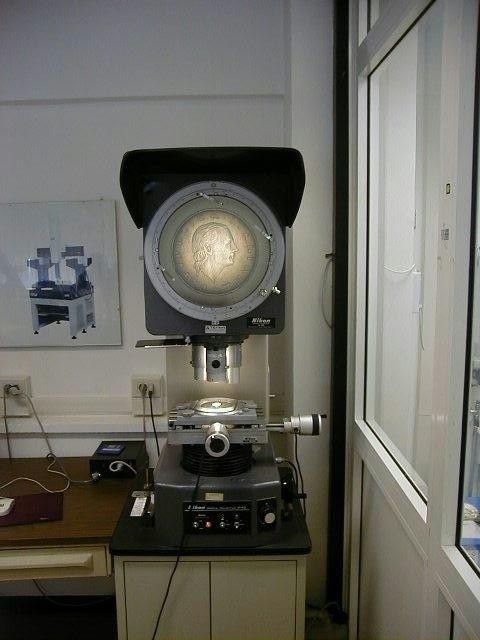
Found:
[97,444,125,455]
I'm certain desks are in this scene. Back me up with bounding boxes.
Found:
[0,457,144,598]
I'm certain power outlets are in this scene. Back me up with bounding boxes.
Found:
[132,374,166,416]
[1,376,32,418]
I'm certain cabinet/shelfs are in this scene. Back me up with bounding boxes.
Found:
[112,554,307,639]
